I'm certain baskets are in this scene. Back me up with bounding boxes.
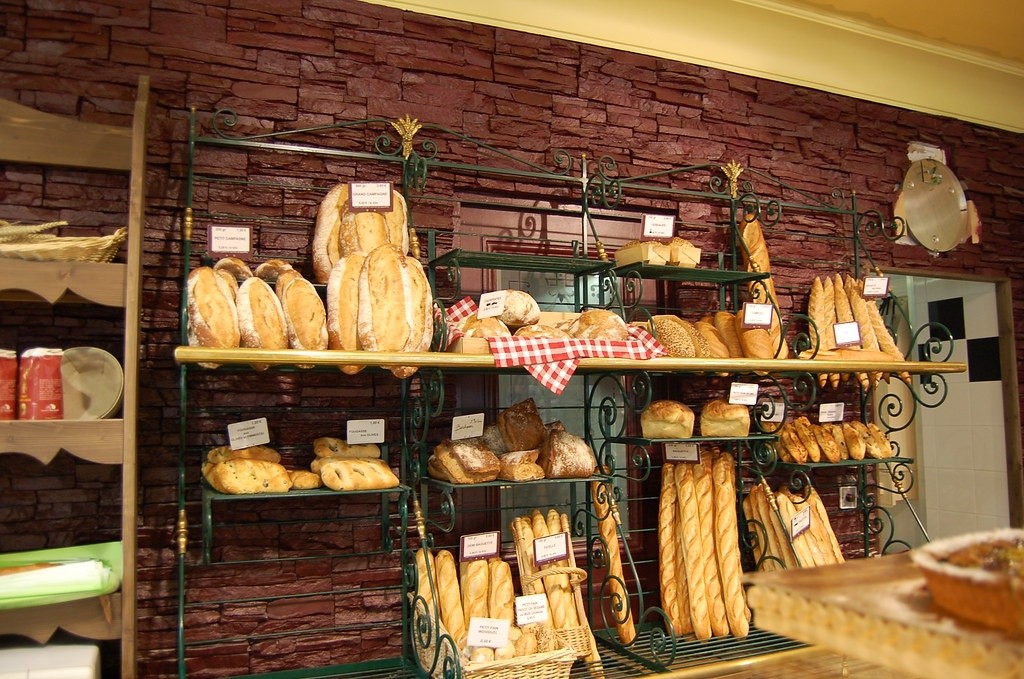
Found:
[556,625,593,657]
[407,590,577,679]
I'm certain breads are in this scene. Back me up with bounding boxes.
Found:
[760,415,893,465]
[413,506,605,679]
[700,398,751,438]
[640,399,695,440]
[591,465,636,648]
[427,395,594,485]
[201,436,400,494]
[180,180,433,380]
[454,214,913,390]
[658,441,845,644]
[0,561,65,578]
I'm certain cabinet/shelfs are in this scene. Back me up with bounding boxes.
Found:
[172,105,969,679]
[0,72,152,679]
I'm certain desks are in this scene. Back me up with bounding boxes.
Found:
[737,552,1024,679]
